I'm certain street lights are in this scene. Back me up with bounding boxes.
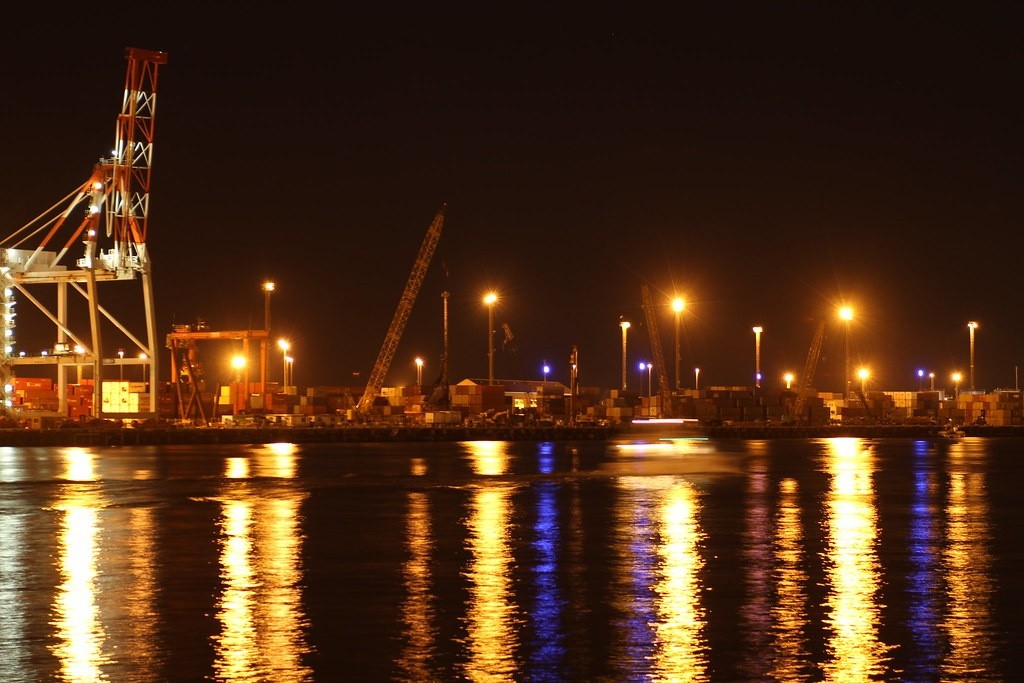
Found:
[696,369,699,389]
[640,363,644,396]
[672,299,683,391]
[264,282,274,381]
[621,322,630,389]
[280,341,293,393]
[486,295,495,385]
[543,361,548,383]
[919,370,934,391]
[753,326,762,387]
[416,359,422,384]
[647,364,652,397]
[842,309,851,399]
[953,375,959,399]
[786,375,791,388]
[968,322,978,391]
[860,371,866,392]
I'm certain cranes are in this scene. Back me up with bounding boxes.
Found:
[640,283,672,417]
[793,319,825,416]
[358,202,449,415]
